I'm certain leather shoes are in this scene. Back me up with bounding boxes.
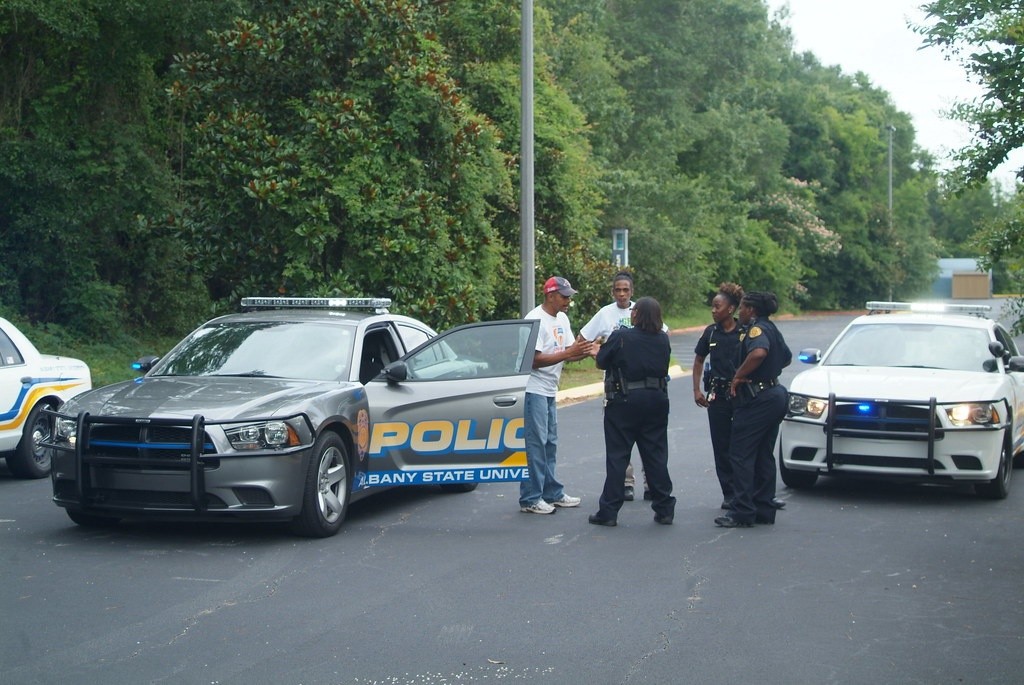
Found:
[653,513,672,524]
[589,514,617,526]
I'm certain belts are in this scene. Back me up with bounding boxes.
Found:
[754,378,780,392]
[626,378,661,389]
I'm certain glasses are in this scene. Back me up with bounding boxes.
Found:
[628,308,636,312]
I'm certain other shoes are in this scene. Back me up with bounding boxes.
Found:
[769,496,786,508]
[623,487,633,501]
[720,501,732,509]
[644,491,652,500]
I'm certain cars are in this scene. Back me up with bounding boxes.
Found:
[0,316,92,476]
[39,295,542,537]
[778,300,1024,500]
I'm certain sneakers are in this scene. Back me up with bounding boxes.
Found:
[547,494,580,507]
[521,499,556,514]
[714,514,755,527]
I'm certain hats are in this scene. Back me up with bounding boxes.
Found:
[544,276,579,296]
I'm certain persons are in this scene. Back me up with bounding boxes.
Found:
[694,282,786,509]
[515,277,602,514]
[589,296,676,527]
[714,292,793,527]
[579,273,671,501]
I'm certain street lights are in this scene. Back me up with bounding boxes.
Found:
[883,123,899,303]
